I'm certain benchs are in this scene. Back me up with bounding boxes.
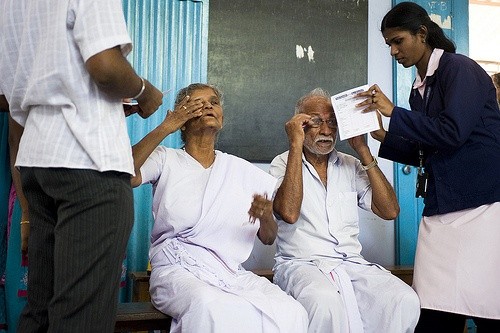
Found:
[112,303,171,333]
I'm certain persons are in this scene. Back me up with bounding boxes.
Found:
[0,0,163,333]
[130,84,309,333]
[268,88,420,333]
[355,2,500,333]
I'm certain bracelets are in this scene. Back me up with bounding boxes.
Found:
[131,76,145,100]
[20,221,30,225]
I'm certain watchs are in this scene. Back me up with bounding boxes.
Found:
[360,156,378,171]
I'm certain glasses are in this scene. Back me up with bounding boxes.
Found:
[310,118,338,129]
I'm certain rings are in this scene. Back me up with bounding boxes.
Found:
[186,109,189,113]
[183,106,186,109]
[372,94,375,98]
[372,98,374,104]
[260,210,264,216]
